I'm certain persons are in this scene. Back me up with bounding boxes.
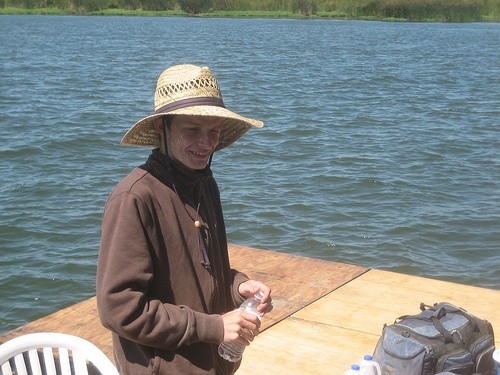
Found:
[94,64,274,375]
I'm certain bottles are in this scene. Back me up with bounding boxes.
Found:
[356,355,381,375]
[217,291,266,362]
[342,364,368,375]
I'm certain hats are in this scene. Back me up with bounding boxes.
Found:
[120,64,264,151]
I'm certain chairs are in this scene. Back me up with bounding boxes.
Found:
[0,332,120,375]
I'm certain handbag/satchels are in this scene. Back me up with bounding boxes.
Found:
[372,301,495,374]
